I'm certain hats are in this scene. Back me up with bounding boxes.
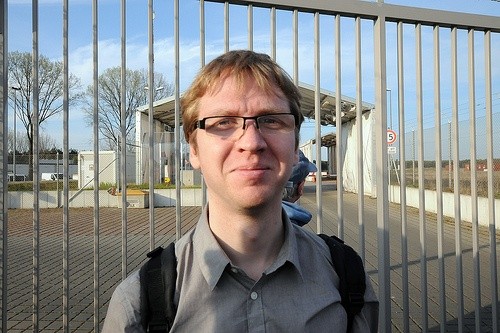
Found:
[289,149,318,180]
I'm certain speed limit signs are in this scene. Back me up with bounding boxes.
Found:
[387,130,397,143]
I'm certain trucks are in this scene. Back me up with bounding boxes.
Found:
[41,172,73,182]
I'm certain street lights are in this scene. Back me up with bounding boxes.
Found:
[386,89,392,166]
[12,86,22,182]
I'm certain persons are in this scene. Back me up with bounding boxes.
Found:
[281,149,316,227]
[102,49,379,333]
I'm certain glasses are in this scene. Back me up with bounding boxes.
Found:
[196,112,300,138]
